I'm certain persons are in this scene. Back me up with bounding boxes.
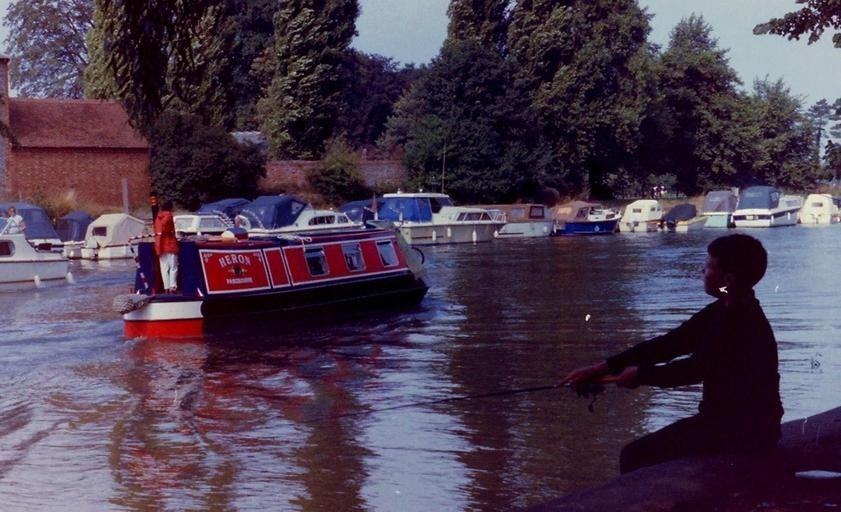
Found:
[152,200,180,295]
[556,232,789,476]
[4,206,26,235]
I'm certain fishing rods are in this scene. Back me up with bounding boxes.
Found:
[248,375,619,431]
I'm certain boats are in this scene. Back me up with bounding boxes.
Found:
[0,218,70,283]
[392,206,508,246]
[0,184,458,262]
[550,200,622,236]
[655,203,701,233]
[120,215,434,341]
[798,193,841,224]
[491,203,553,238]
[617,199,666,232]
[700,190,739,228]
[731,185,804,227]
